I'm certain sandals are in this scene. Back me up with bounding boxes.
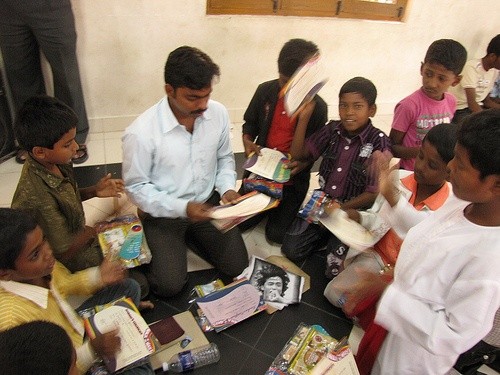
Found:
[16,150,28,163]
[73,145,87,163]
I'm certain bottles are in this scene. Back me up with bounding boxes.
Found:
[162,342,220,374]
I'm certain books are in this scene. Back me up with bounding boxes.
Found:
[80,296,161,373]
[95,213,152,268]
[146,310,211,370]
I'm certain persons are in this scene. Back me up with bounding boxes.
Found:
[121,46,250,297]
[0,207,155,375]
[255,264,293,303]
[0,320,79,375]
[241,39,328,244]
[281,77,392,276]
[446,33,500,121]
[355,107,500,375]
[390,38,467,170]
[344,122,455,276]
[9,95,154,309]
[0,0,89,164]
